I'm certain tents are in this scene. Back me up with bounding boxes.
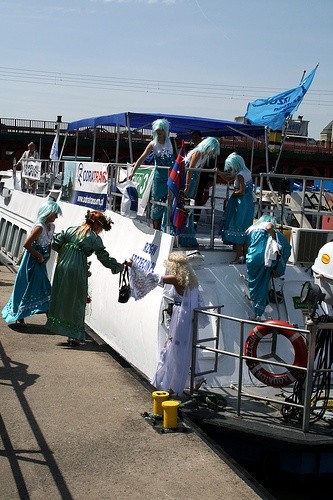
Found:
[67,112,265,173]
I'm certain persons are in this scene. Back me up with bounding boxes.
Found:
[128,119,179,238]
[245,214,282,320]
[1,201,62,328]
[125,251,210,397]
[46,210,124,346]
[13,142,39,194]
[161,137,220,249]
[213,152,257,265]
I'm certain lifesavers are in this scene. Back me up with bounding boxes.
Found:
[244,320,308,388]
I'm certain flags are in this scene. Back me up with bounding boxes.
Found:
[49,133,58,176]
[167,140,186,236]
[245,65,318,132]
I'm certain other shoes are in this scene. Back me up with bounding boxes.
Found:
[252,315,261,321]
[246,289,252,300]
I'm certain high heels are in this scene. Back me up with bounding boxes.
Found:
[231,255,245,264]
[16,318,27,327]
[67,337,85,345]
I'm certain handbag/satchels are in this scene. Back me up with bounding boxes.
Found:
[118,262,131,303]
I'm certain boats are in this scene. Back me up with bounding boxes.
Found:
[203,174,333,328]
[0,159,333,445]
